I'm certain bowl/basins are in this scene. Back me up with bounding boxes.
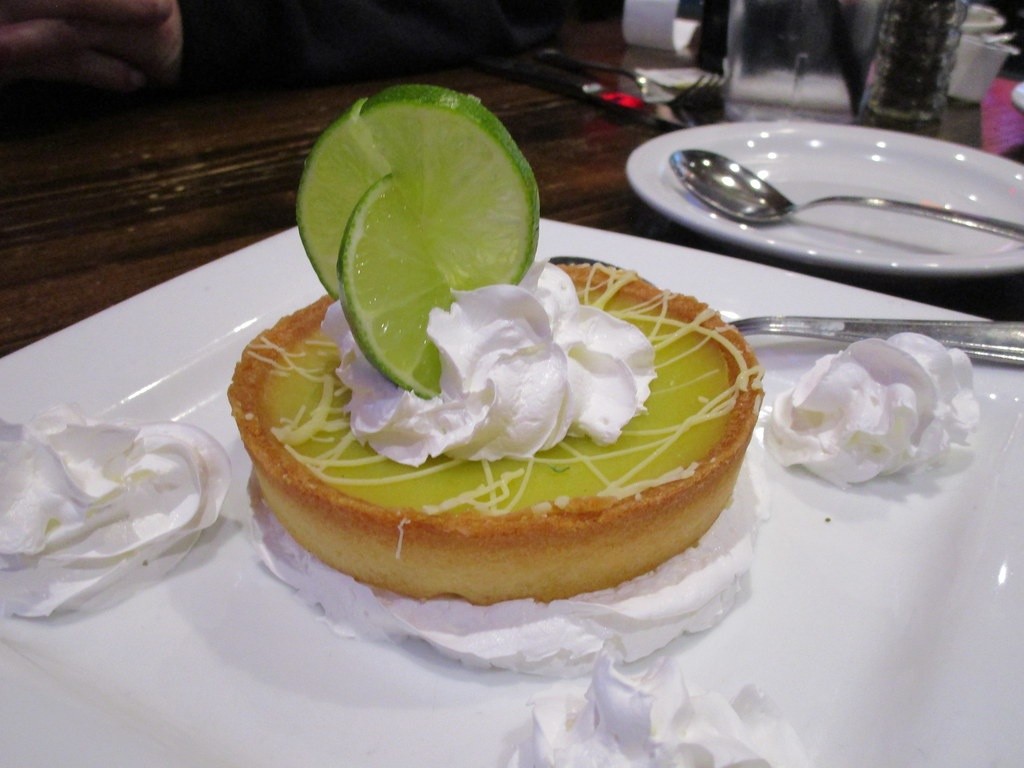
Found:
[853,0,1005,62]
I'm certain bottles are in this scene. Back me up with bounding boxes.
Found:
[861,0,967,140]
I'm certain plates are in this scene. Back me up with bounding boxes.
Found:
[0,216,1024,768]
[624,121,1024,275]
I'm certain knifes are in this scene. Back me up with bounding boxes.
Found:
[416,44,707,131]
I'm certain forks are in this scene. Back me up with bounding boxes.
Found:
[530,52,729,106]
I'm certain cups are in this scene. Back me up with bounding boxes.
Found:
[727,0,881,122]
[949,34,1017,102]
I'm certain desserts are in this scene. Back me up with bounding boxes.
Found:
[223,84,766,677]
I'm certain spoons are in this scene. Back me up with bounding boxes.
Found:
[665,146,1024,246]
[546,254,1024,364]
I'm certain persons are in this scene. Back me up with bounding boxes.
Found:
[0,0,571,108]
